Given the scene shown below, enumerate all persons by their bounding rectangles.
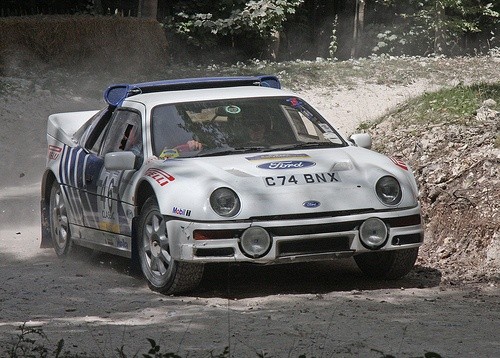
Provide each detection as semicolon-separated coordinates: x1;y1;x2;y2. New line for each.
229;106;275;151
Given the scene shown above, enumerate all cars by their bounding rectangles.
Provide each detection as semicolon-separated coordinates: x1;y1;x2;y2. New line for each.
40;77;425;295
